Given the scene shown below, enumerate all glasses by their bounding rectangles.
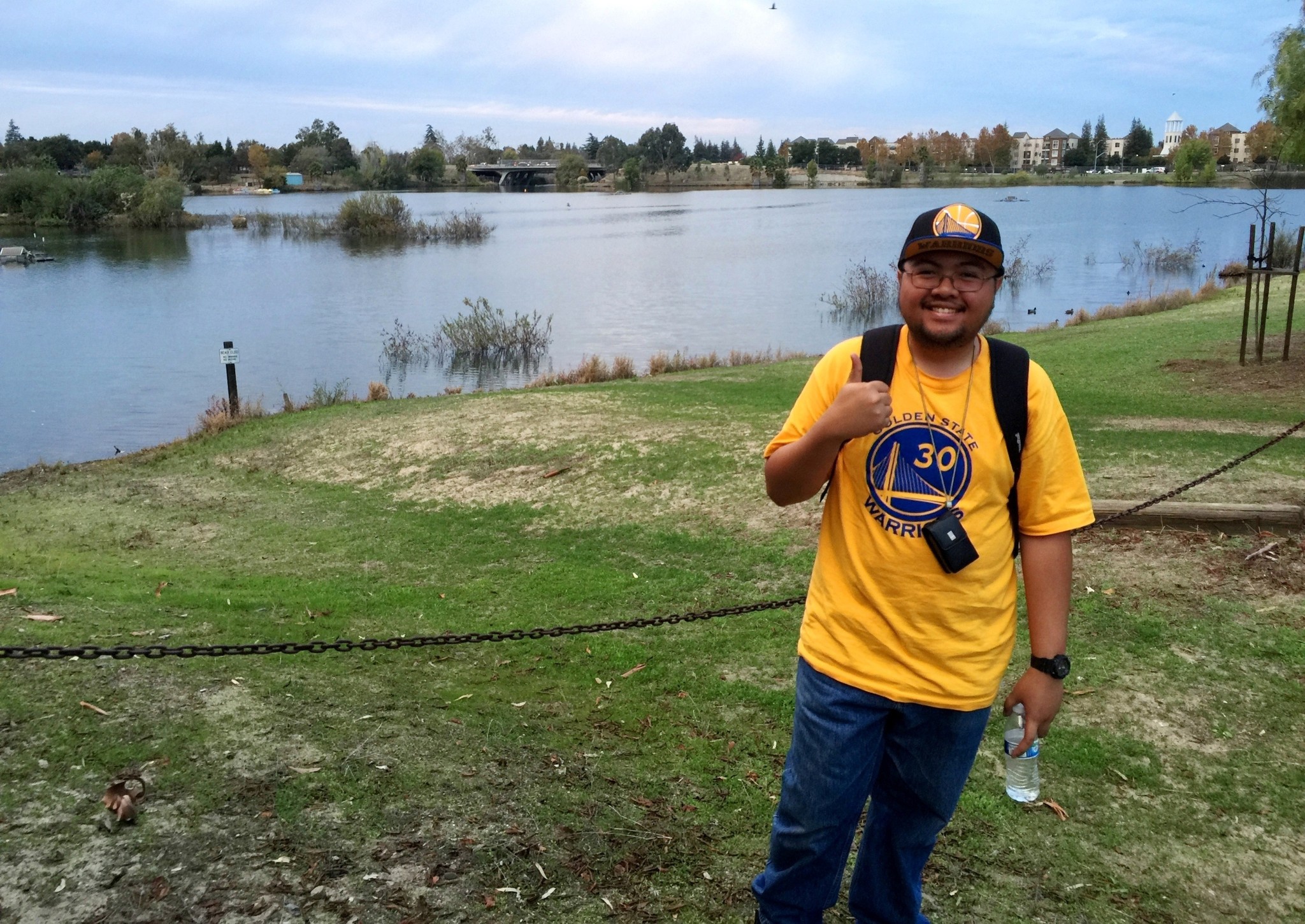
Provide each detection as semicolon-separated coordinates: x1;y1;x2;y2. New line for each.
902;261;999;292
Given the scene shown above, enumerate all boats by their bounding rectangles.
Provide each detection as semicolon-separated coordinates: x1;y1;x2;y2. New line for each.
231;188;280;194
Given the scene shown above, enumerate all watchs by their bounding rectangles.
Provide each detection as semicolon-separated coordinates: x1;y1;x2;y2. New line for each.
1030;652;1071;680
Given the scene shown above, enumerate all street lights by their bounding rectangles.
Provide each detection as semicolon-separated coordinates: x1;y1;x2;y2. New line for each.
1094;139;1103;170
1264;145;1267;168
497;141;499;160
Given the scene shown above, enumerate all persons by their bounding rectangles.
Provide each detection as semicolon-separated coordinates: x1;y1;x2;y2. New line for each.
750;203;1096;924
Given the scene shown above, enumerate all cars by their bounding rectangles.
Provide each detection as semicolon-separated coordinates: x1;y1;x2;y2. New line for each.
1096;169;1120;174
1086;169;1097;173
1243;167;1267;172
518;162;530;166
480;162;486;165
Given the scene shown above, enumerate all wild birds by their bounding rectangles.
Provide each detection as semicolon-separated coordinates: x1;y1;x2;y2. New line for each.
1028;307;1036;312
769;3;777;9
1202;264;1205;267
1066;309;1073;313
1127;291;1130;293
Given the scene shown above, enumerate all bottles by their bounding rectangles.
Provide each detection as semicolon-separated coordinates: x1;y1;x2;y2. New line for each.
1003;703;1040;802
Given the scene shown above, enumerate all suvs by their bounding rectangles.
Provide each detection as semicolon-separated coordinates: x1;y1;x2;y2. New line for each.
1146;167;1163;174
537;162;550;166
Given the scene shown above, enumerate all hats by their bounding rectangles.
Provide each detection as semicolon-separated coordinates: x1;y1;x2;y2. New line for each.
897;203;1004;272
